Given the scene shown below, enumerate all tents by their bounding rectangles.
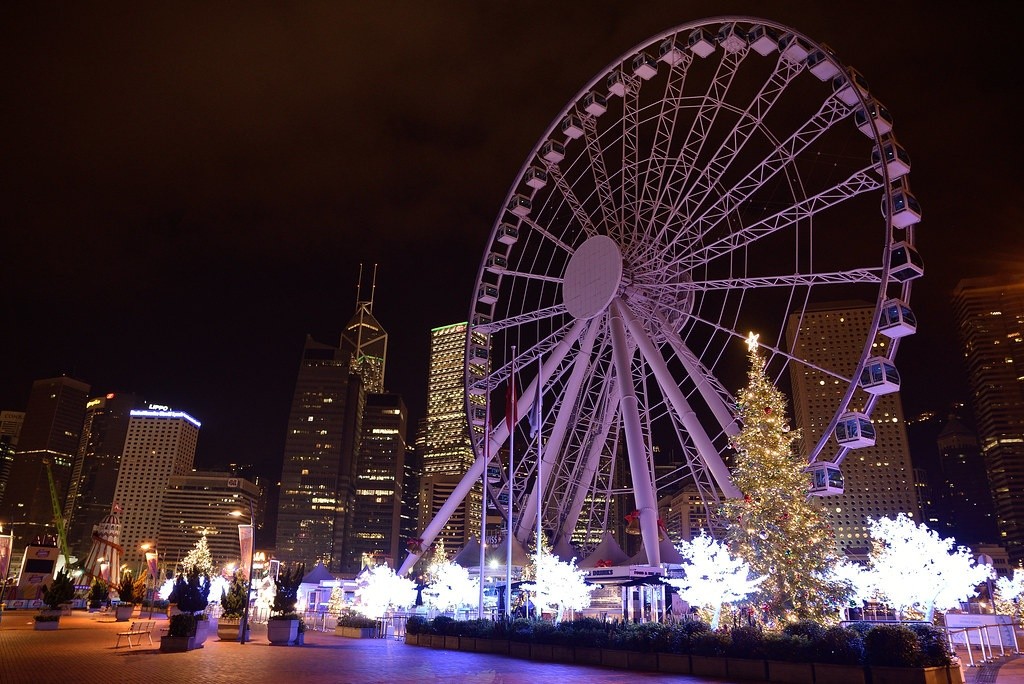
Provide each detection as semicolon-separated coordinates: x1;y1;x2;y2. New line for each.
290;532;692;627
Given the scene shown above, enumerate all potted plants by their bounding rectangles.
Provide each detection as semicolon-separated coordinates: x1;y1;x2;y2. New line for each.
33;562;379;652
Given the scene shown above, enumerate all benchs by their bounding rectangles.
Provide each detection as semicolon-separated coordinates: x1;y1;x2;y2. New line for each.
115;620;156;649
